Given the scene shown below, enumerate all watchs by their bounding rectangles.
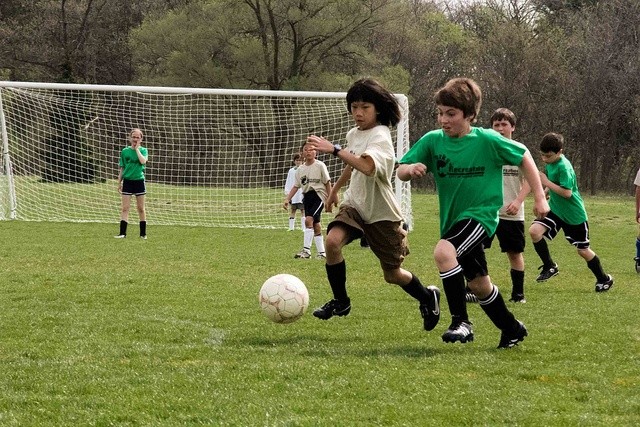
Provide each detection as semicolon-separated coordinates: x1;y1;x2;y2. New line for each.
333;144;341;156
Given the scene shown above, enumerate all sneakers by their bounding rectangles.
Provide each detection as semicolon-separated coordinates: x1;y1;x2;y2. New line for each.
114;235;125;238
497;319;528;348
509;292;526;303
316;253;326;258
442;315;473;342
536;262;560;282
295;249;311;259
313;298;351;319
634;257;640;272
140;234;147;239
419;285;440;331
595;274;613;292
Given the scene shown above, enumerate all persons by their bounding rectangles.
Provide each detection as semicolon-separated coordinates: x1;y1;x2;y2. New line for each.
396;79;551;349
283;142;333;260
529;132;614;292
465;108;532;302
312;77;441;331
284;153;306;232
634;168;640;273
113;128;149;240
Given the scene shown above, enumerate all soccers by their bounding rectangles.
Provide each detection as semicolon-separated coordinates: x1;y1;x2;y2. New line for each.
258;274;309;323
